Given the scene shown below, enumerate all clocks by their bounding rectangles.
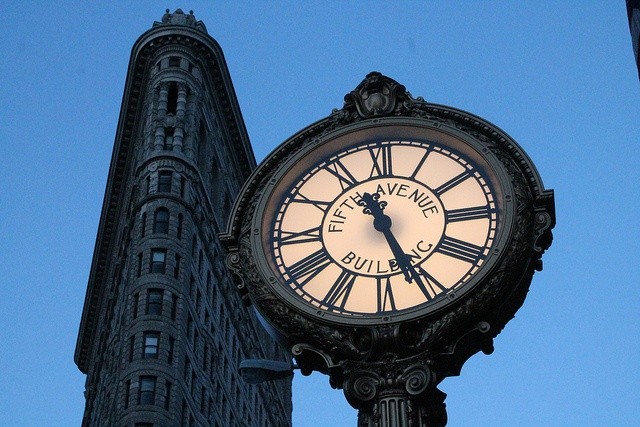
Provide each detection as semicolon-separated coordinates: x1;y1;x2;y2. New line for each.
251;114;518;326
